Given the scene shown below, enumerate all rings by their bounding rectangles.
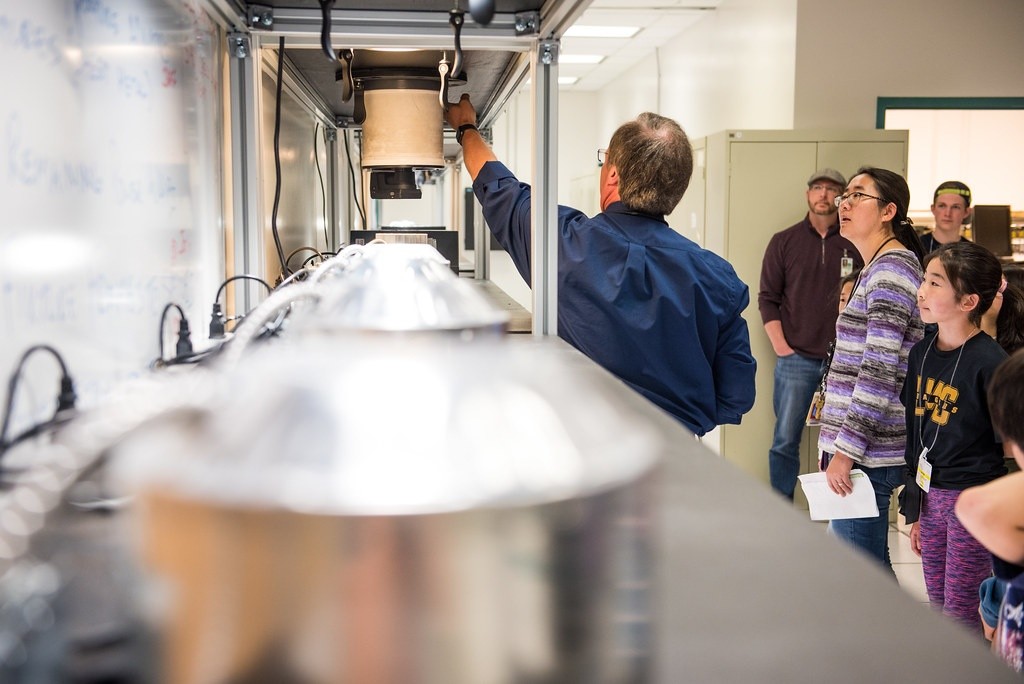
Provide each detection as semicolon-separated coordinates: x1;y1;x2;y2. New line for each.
839;483;842;485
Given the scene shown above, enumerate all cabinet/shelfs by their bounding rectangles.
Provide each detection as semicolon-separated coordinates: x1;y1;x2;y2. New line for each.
665;129;910;512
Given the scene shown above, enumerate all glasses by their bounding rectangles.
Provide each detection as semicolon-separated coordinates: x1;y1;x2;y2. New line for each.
834;192;889;207
598;149;609;162
809;184;843;195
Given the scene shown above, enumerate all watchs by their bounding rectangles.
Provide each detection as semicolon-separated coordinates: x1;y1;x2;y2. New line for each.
456;124;477;146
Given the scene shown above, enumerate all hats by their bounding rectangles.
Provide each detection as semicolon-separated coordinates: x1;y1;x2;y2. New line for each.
934;181;971;224
807;168;847;188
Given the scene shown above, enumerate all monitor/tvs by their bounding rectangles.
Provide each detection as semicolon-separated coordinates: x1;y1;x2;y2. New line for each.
971;205;1012;256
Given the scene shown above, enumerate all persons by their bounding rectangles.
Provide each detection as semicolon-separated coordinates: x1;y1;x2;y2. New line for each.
816;167;925;585
919;181;972;255
951;346;1024;674
758;167;865;505
898;242;1024;648
443;93;758;438
